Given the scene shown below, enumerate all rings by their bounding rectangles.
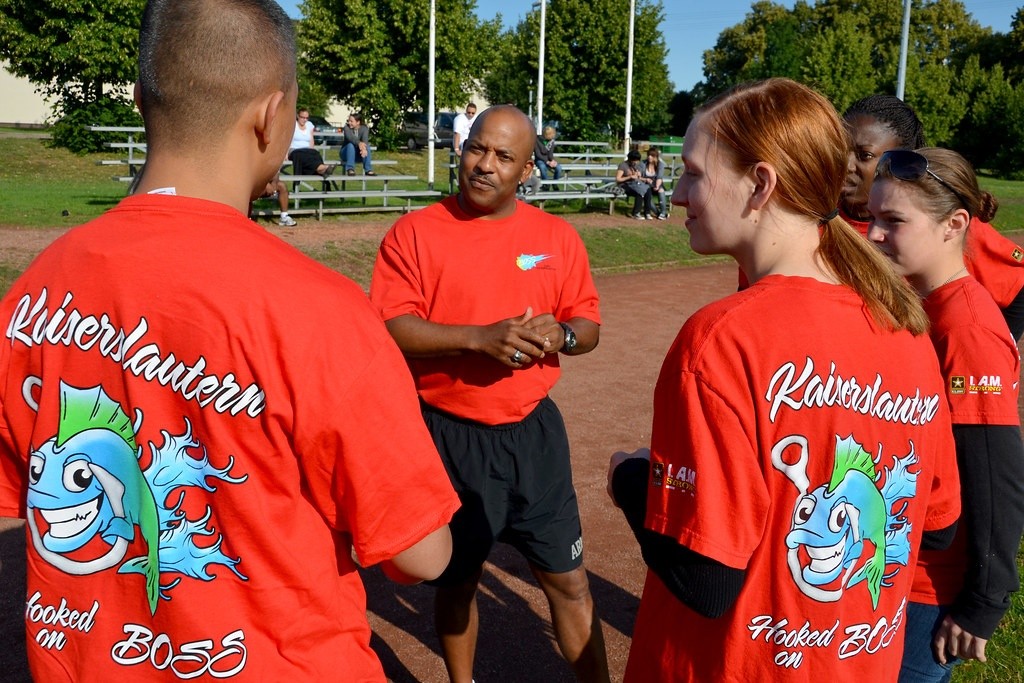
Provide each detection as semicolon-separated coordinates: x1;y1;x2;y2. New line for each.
514;351;522;362
544;335;549;342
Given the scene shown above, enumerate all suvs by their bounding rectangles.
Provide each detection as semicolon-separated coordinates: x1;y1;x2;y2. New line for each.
397;112;460;150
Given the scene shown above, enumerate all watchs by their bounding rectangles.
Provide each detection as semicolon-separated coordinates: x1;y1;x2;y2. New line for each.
559;322;577;353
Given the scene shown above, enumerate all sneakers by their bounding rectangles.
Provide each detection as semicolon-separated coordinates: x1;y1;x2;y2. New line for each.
279;215;297;226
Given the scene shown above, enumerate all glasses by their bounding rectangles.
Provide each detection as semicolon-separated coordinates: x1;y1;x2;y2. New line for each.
467;112;475;114
299;115;309;119
876;150;972;218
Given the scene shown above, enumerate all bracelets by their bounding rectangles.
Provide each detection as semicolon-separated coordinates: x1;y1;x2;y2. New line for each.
454;148;459;151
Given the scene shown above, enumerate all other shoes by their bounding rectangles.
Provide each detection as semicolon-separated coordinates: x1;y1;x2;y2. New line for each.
646;213;653;219
633;213;645;220
347;169;356;176
324;168;331;179
553;185;559;191
543;185;550;191
666;213;670;218
330;163;337;174
658;213;666;220
365;171;377;176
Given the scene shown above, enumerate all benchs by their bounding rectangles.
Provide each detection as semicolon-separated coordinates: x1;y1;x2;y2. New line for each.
88;123;684;222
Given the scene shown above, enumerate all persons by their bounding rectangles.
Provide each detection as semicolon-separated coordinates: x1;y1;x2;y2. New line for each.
1;0;612;683
288;107;336;193
606;77;1024;682
616;149;653;220
259;172;297;226
642;146;669;220
340;112;377;176
453;103;478;156
534;126;562;191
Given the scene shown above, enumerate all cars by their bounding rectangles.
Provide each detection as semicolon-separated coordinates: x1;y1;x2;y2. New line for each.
307;116;345;144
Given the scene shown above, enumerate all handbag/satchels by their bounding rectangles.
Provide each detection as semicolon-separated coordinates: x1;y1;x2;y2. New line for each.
628;179;650;198
